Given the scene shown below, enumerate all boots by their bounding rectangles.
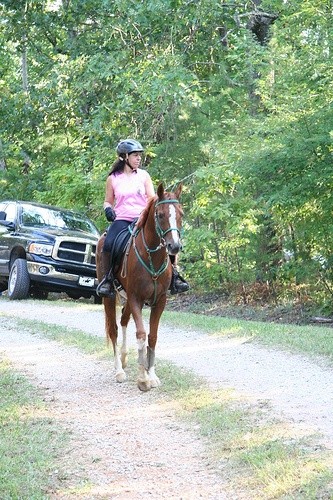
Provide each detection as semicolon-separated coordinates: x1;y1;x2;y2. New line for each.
169;254;189;294
99;252;111;295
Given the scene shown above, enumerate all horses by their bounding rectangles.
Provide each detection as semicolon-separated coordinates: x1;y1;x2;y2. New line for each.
96;183;184;391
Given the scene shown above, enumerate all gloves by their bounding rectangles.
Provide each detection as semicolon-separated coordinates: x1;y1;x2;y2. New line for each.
105;207;116;222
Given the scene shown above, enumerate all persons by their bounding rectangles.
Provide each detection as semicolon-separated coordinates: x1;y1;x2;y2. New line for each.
98;140;189;295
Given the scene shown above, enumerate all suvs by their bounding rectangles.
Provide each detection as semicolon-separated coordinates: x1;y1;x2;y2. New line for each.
0;200;106;305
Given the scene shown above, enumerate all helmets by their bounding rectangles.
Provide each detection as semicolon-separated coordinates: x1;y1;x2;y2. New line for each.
116;139;145;155
